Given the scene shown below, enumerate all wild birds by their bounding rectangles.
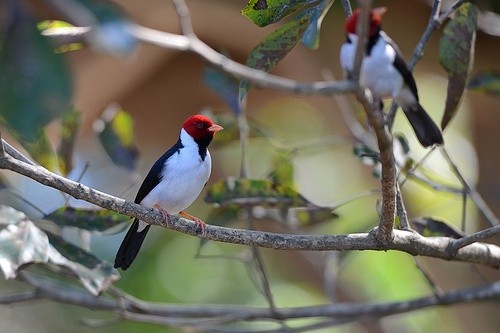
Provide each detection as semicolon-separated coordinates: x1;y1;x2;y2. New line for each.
113;113;224;271
337;6;445;146
438;2;479;131
